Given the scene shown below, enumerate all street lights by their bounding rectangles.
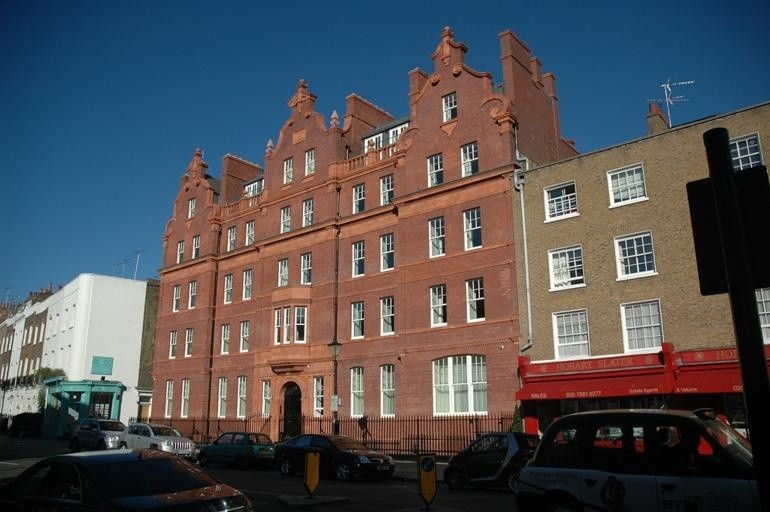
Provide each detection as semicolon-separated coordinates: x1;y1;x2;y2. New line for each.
328;336;341;436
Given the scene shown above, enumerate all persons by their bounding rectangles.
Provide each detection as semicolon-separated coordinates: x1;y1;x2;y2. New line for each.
359;411;372;444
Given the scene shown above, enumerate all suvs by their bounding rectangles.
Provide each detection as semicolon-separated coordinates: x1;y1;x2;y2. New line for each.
70;419;126;454
121;421;195;458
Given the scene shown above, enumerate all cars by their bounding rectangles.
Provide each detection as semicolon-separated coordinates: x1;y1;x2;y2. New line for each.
732;420;749;440
0;448;253;511
516;407;759;511
271;431;394;480
445;432;538;491
195;431;277;467
